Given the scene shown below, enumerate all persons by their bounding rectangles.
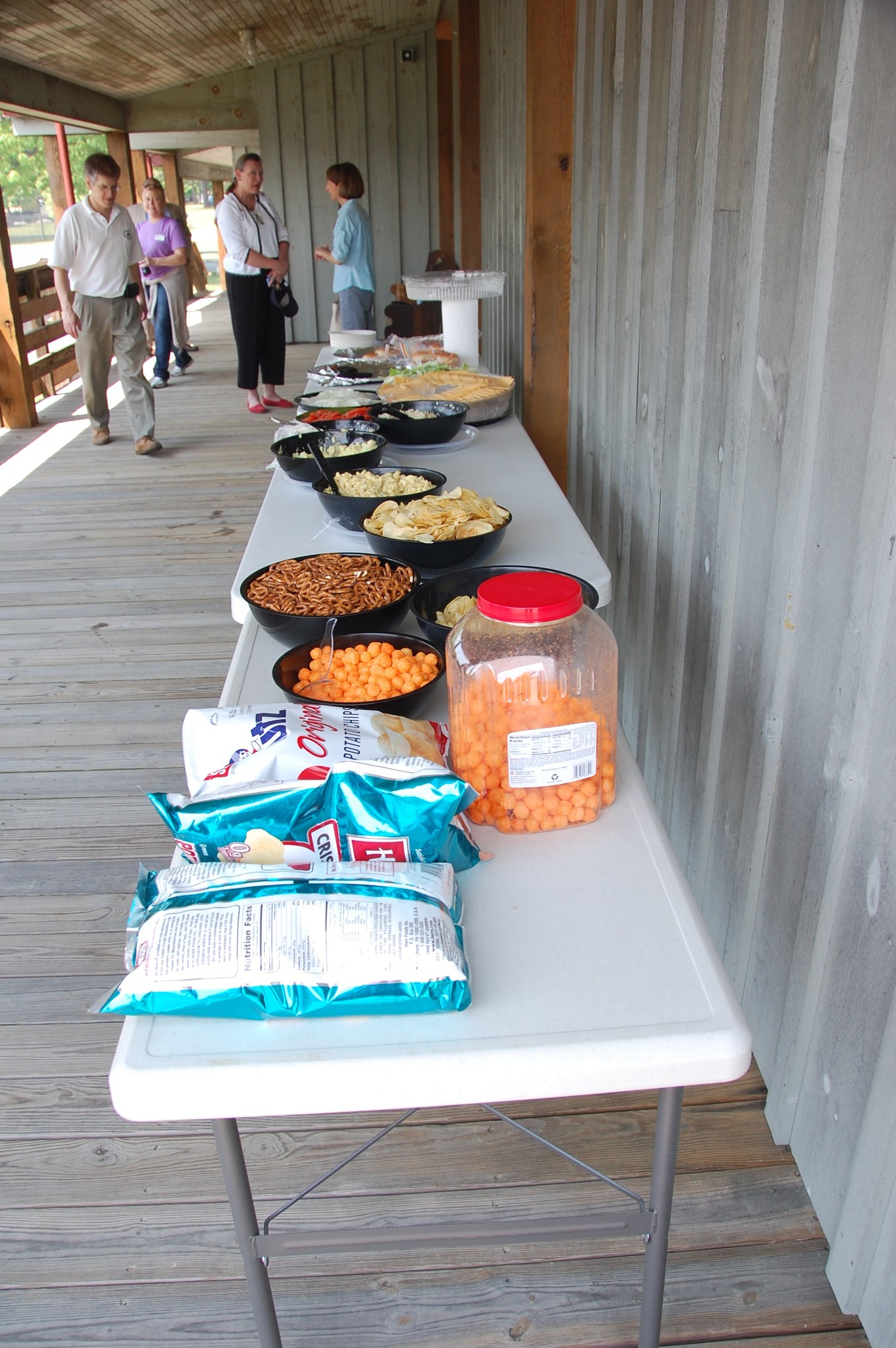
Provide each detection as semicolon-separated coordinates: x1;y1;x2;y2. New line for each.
214;176;238;252
133;185;196;391
47;152;165;454
313;160;378;341
217;152;295;413
125;177;199;356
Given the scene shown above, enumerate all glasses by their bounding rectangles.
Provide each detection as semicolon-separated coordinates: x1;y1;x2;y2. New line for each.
91;181;124;193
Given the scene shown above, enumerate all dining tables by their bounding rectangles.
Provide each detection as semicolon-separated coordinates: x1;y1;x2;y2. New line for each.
226;333;610;614
107;606;749;1348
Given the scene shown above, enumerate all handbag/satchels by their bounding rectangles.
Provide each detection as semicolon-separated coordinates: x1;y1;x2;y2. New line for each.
329;299;342;334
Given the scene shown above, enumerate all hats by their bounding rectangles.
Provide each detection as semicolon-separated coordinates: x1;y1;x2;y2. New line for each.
270;278;300;318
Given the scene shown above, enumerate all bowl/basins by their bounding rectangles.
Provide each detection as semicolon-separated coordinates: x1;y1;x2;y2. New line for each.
239;552;599;718
328;330;376;349
270;390;513;583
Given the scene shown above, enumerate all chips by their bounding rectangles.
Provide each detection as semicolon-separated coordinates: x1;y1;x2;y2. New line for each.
86;697;495;1030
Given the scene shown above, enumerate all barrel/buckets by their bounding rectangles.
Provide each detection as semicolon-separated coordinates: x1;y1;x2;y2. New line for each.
445;570;618;832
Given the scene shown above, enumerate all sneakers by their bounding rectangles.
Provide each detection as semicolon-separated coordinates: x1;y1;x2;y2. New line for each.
172;358;194;375
150;375;167;389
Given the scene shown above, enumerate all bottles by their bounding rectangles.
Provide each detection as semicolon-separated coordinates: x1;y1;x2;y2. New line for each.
141;267;151;276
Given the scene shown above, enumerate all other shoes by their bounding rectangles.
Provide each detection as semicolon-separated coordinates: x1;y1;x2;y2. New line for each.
247;398;266;413
262;393;293;408
92;425;110;445
148;347;156;357
135;436;162;456
180;342;197;351
